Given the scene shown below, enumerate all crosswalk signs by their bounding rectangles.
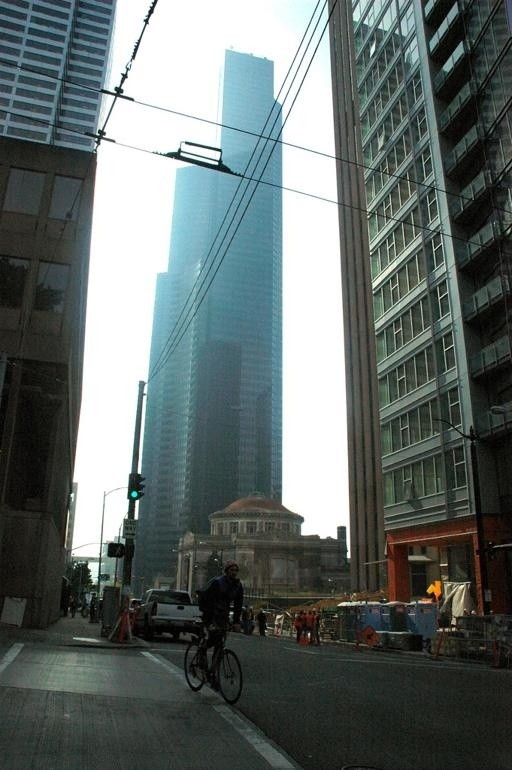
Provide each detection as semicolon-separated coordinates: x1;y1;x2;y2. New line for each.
108;543;124;558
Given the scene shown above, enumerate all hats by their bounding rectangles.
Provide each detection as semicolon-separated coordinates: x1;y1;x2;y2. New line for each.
224;559;240;574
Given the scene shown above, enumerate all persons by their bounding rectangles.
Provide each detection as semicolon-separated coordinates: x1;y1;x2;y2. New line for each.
241;605;321;647
68;592;99;625
195;558;245;691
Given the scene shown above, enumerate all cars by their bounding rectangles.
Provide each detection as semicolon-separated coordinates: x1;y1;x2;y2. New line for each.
229;606;257;632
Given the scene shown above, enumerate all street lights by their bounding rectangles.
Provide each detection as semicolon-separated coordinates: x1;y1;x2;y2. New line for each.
432;418;491;615
172;541;223;591
327;576;336;593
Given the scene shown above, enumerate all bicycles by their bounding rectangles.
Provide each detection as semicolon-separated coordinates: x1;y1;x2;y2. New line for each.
184;622;243;705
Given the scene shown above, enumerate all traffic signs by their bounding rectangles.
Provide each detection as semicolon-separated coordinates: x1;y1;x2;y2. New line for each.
122;519;137;539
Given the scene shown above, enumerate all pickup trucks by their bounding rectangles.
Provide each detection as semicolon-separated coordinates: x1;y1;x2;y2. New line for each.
129;589;204;640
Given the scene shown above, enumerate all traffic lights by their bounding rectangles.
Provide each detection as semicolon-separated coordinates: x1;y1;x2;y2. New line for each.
128;473;146;500
487;540;496;562
